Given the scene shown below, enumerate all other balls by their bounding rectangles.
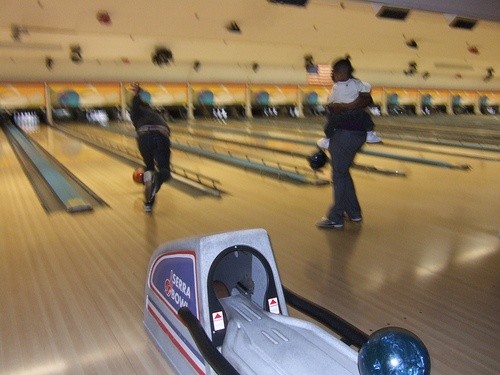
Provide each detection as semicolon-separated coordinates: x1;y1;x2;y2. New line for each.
133;168;145;185
357;326;431;375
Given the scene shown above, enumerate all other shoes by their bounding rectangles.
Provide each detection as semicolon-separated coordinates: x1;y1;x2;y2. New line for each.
366;130;382;143
316;137;330;149
143;170;157;202
144;205;152;212
344;211;363;221
317;219;343;229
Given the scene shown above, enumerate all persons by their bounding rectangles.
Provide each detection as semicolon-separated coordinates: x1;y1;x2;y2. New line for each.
127;81;174;212
317;59;382;150
316;55;374;228
